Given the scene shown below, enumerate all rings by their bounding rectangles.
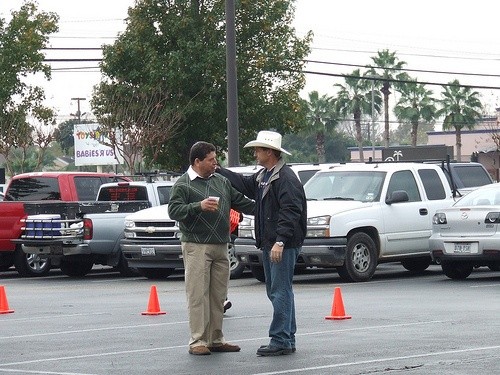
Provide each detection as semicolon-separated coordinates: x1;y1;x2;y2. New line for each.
275;255;279;258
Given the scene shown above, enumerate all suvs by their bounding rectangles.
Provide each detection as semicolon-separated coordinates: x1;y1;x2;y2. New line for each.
119;162;367;279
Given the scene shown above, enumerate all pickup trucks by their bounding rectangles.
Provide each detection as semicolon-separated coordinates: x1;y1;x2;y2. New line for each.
232;158;495;283
0;172;138;278
11;170;176;278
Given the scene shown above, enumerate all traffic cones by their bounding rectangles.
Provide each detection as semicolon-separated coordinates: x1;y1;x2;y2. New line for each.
0;285;15;314
324;287;351;320
141;285;166;316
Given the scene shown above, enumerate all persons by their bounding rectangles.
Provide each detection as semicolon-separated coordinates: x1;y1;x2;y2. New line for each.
168;141;257;355
213;130;307;355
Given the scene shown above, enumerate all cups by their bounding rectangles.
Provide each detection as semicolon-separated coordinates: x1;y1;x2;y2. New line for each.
209;196;219;208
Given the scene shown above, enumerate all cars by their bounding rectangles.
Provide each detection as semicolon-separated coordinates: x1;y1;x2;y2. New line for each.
428;184;500;280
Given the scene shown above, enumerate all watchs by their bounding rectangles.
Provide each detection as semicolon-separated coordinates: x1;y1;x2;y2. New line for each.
275;241;285;247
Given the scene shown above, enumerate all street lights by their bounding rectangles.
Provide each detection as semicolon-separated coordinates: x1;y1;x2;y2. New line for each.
364;74;382;162
71;98;87;124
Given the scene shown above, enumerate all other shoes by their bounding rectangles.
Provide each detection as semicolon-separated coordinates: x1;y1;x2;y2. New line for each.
188;345;211;355
207;343;241;352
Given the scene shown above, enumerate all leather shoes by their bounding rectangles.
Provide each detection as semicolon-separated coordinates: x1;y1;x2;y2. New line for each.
256;344;296;356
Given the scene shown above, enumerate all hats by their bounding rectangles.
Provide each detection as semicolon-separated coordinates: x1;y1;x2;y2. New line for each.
243;130;293;156
